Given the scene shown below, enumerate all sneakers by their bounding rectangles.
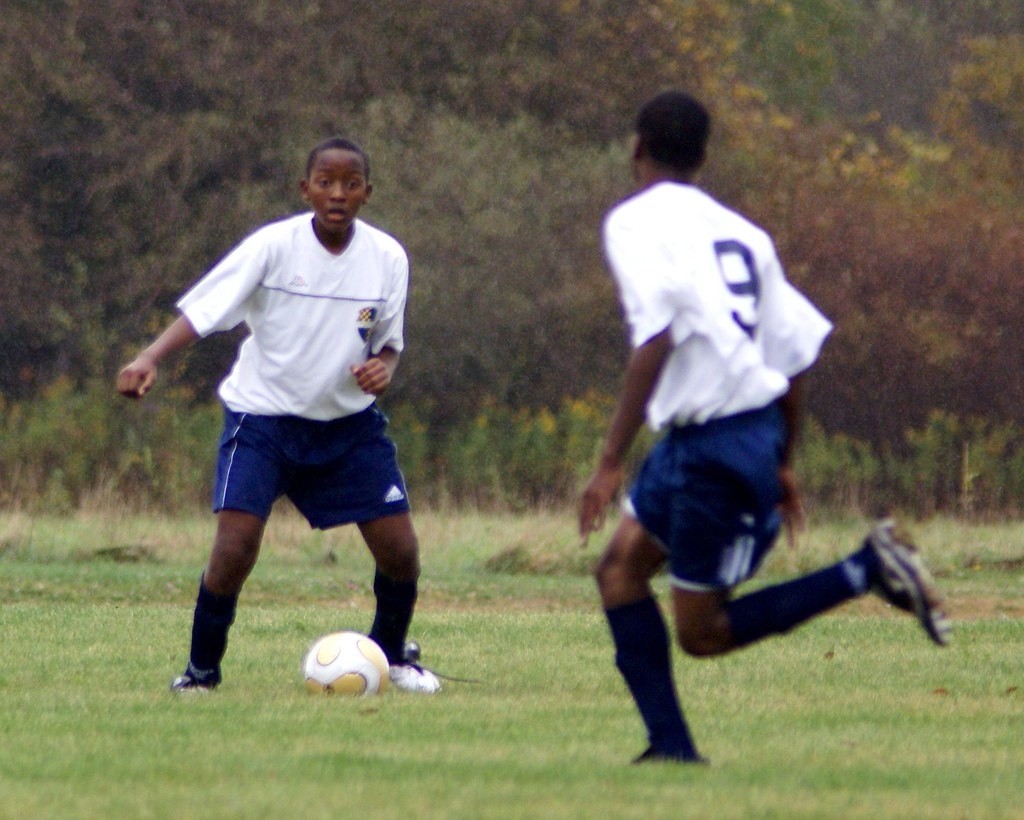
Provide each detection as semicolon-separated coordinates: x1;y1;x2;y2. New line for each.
864;519;955;646
388;659;439;692
171;663;219;690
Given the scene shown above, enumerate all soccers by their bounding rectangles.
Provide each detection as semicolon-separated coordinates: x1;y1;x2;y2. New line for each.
305;631;389;699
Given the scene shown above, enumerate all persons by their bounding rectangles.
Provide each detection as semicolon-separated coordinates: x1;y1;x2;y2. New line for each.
576;94;954;762
117;139;441;692
